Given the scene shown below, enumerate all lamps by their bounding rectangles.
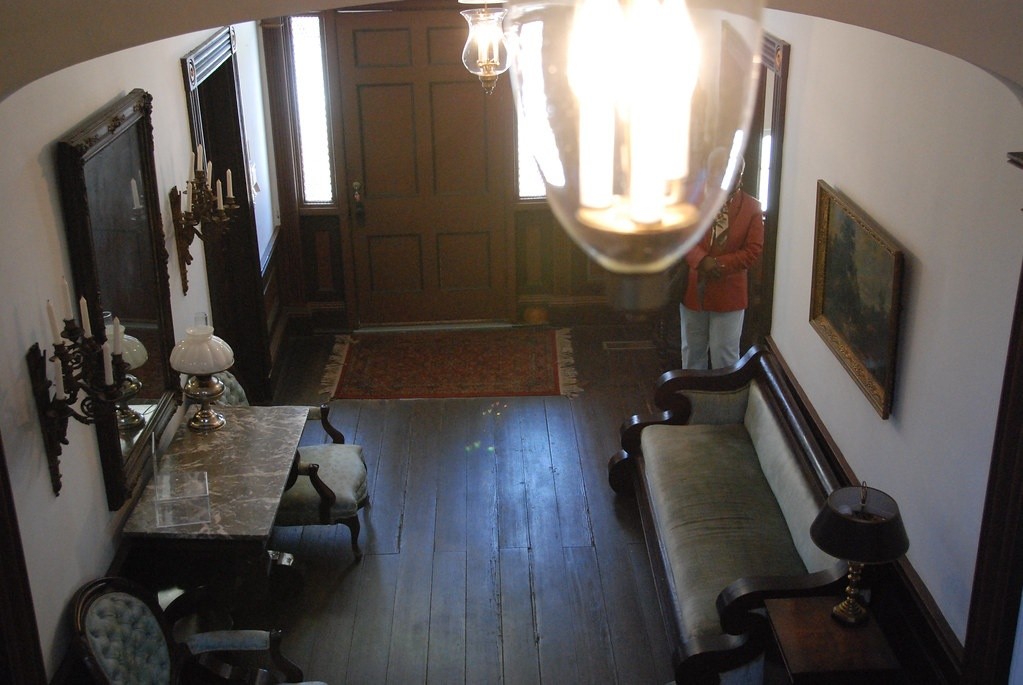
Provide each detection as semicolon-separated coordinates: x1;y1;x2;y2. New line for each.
169;322;236;433
808;481;911;629
100;312;148;431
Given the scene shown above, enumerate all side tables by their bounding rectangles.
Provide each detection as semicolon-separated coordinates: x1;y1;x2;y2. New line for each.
758;592;908;684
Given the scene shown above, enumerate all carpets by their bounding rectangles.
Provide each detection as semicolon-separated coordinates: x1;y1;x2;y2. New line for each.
315;325;579;404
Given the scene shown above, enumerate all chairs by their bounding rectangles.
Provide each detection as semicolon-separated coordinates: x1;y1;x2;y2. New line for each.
178;370;370;554
68;574;327;685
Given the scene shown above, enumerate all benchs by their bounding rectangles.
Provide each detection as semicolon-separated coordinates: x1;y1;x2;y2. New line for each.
608;348;892;685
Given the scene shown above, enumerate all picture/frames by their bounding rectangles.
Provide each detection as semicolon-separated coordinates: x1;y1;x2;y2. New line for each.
806;179;904;421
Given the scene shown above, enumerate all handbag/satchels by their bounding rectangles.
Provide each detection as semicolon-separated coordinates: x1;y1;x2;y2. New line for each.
665;257;690;315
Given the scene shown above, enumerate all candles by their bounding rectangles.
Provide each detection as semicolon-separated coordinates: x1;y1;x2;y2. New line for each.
55;356;65;400
188;143;213;192
80;295;92;338
226;168;233;198
112;315;122;355
101;338;114;385
216;179;224;210
186;181;193;212
131;176;140;208
47;298;62;344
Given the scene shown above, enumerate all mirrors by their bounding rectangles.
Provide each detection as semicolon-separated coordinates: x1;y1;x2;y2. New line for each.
48;86;171;344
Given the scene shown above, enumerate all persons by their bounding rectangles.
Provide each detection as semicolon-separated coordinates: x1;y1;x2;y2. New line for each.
679;147;764;369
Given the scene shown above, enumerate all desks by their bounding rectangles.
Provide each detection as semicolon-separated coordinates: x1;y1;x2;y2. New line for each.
117;402;311;614
